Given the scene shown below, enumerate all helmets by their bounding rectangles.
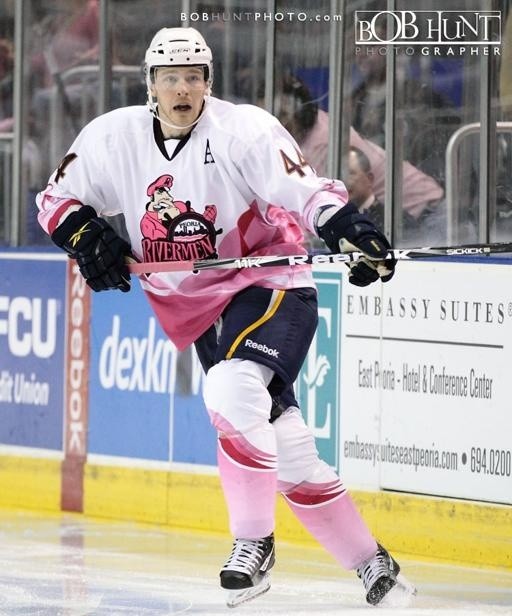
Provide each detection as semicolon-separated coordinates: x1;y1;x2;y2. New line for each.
141;28;216;101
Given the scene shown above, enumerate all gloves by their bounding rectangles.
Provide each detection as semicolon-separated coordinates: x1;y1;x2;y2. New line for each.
316;202;396;288
50;204;136;292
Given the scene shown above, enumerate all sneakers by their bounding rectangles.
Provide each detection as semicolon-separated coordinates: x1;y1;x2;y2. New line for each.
219;533;276;588
355;543;401;607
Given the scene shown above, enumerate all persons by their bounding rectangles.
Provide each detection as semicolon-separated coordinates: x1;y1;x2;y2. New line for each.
0;0;477;251
35;25;400;606
